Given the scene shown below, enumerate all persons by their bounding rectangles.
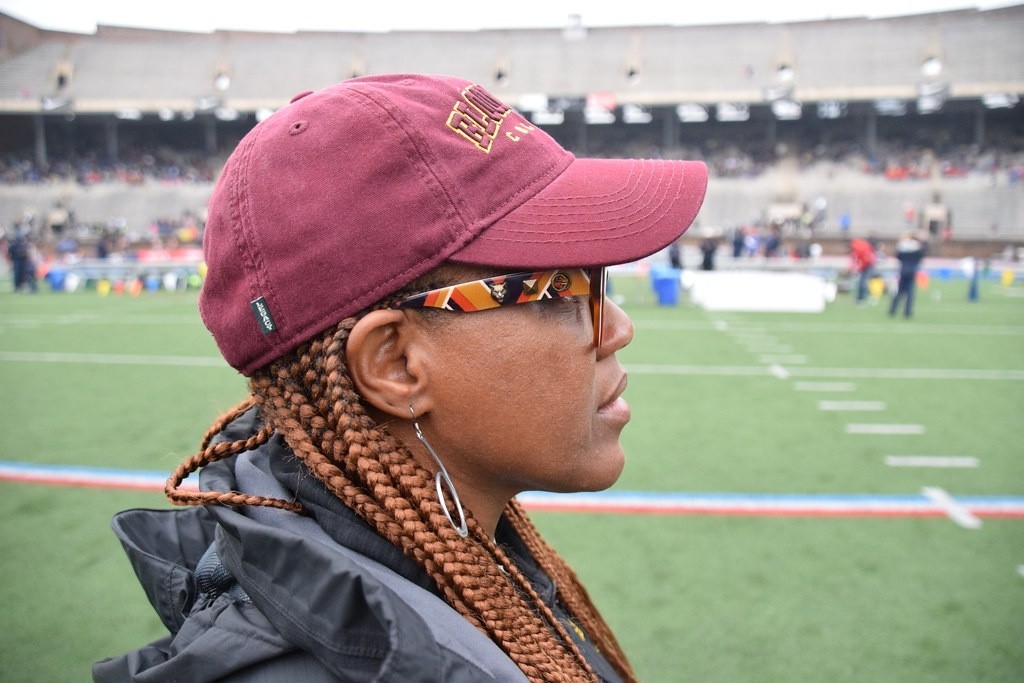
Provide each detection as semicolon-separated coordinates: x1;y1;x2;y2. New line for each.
0;120;1024;323
87;68;641;683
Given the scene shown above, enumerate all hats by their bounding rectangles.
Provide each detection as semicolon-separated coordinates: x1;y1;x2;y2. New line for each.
197;75;708;377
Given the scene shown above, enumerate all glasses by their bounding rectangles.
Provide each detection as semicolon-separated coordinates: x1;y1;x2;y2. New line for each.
388;267;606;345
